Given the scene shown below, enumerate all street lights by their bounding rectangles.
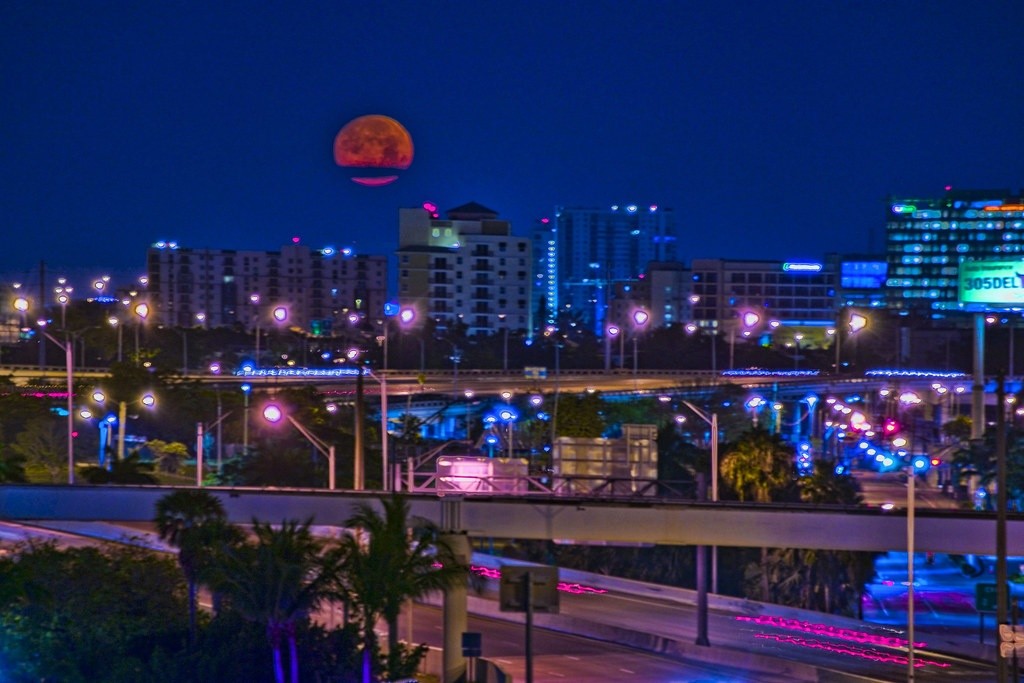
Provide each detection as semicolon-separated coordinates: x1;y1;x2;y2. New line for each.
383;308;416;492
264;404;339;489
196;405;259;486
659;393;718;594
18;322;77;486
93;390;155;462
